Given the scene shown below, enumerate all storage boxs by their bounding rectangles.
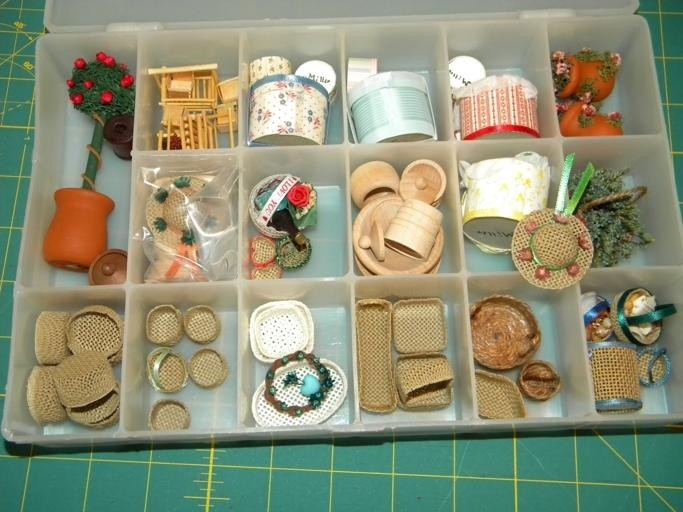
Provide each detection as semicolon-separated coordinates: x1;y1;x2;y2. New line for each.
1;0;682;447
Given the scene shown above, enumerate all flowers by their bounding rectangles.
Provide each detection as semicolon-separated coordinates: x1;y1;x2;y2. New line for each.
287;183;312;210
549;45;622;104
552;100;624;131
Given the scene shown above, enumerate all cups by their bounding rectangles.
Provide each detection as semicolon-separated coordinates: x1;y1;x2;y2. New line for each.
382;199;444;262
87;249;126;285
102;114;132;160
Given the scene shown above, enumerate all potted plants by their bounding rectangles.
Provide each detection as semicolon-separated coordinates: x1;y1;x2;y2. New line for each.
39;50;136;272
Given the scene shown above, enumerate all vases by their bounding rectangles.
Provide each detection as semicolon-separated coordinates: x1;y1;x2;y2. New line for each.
556;100;623;137
555;53;616;104
